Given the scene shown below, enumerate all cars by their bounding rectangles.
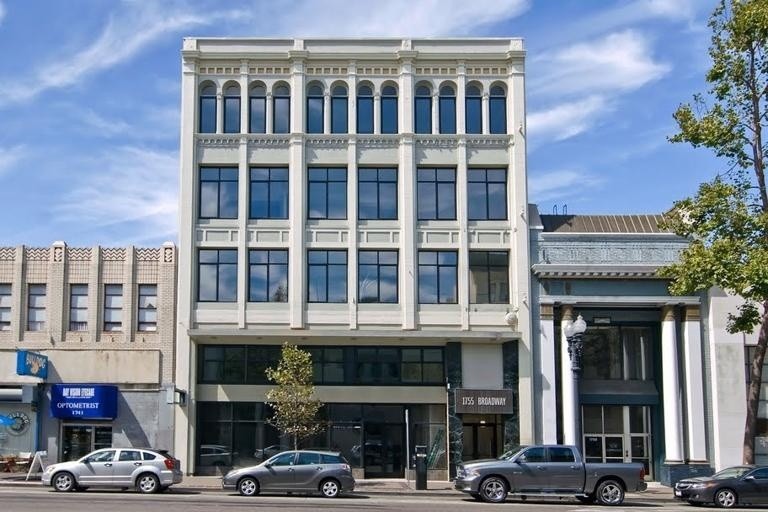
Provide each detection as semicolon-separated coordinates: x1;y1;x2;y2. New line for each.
199;445;240;474
41;447;183;494
674;464;768;508
413;445;462;467
254;444;289;459
352;443;393;465
222;450;355;498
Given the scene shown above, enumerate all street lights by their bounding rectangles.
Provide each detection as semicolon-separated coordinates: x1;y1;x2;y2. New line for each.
564;312;587;445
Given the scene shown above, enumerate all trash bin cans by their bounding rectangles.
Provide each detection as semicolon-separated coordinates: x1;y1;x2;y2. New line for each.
415;445;428;490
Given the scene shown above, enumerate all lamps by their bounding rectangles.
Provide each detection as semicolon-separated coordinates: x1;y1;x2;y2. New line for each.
480;420;485;429
593;316;611;324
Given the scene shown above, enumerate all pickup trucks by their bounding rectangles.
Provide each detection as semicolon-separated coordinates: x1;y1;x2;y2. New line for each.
454;444;647;506
